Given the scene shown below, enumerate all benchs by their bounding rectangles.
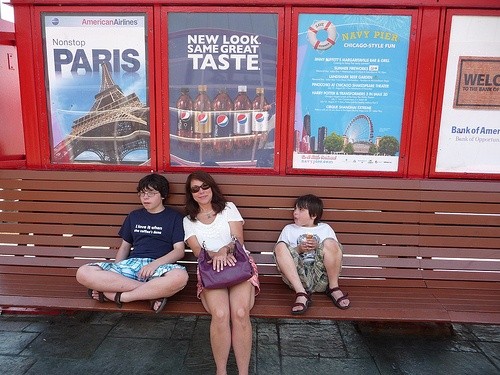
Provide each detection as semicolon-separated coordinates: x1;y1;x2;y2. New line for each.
0;168;500;326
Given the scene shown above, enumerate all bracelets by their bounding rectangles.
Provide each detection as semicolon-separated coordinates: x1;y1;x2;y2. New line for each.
224;245;230;253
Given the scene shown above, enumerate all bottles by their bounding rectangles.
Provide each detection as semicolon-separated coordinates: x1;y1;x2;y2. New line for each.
234;86;252;149
212;87;234;153
251;88;268;135
193;84;212;151
176;88;193;150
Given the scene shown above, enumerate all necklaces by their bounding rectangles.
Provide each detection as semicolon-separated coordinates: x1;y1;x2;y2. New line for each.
205;209;213;219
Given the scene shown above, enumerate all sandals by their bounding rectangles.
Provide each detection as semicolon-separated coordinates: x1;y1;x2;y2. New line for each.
88;288;123;308
291;291;312;315
326;284;351;310
150;297;167;314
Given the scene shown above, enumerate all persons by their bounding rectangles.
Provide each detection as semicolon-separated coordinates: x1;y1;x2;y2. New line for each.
272;194;351;314
183;171;260;375
76;174;189;314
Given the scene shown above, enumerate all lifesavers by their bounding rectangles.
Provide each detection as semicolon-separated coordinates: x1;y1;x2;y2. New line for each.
307;20;336;50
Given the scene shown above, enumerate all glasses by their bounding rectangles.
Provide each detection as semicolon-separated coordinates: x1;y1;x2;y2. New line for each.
191;181;210;193
137;191;158;197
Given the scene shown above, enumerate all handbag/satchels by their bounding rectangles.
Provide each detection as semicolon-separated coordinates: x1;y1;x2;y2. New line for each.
198;238;254;289
